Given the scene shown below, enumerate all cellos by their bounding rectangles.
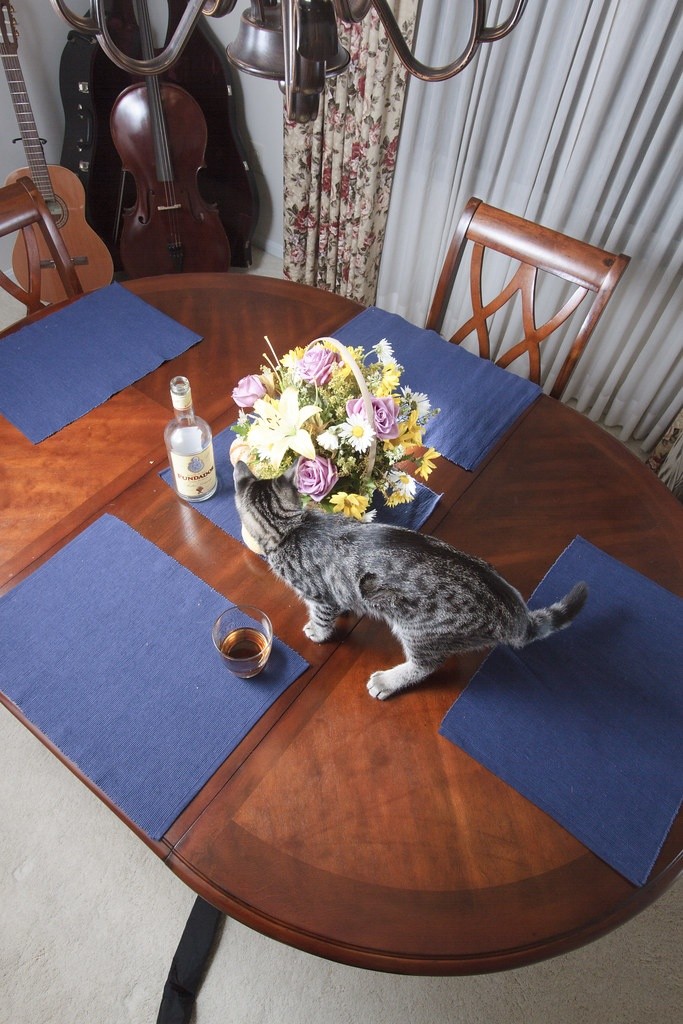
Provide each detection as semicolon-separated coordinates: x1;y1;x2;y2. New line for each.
110;0;231;280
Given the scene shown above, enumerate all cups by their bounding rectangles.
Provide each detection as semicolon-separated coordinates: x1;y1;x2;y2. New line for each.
212;604;273;679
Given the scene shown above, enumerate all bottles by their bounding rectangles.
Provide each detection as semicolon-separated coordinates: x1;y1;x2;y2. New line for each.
163;375;218;503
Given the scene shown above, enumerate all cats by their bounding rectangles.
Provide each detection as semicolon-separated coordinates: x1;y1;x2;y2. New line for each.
232;454;588;701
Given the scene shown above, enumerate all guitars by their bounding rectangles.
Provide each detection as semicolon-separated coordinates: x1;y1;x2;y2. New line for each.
0;0;114;304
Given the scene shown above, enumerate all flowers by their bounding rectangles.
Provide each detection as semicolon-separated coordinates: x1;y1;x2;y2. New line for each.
230;334;441;522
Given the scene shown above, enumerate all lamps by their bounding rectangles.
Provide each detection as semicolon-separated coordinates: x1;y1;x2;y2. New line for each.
49;1;532;127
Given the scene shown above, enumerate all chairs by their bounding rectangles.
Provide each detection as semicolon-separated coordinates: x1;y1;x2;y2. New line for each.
423;195;632;402
0;175;84;333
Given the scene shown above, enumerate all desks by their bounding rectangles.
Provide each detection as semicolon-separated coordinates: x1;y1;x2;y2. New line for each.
0;274;682;1023
